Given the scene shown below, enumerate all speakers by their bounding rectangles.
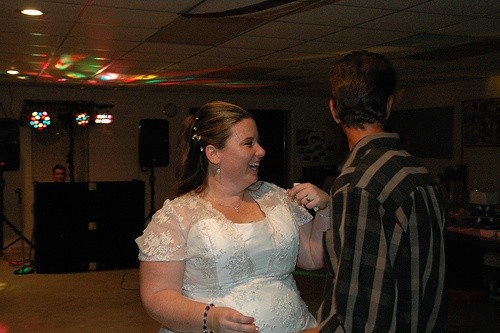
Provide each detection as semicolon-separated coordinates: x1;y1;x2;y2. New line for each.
138;119;170;168
0;117;20;172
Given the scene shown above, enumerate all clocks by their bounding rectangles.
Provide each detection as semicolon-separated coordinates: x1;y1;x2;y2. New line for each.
164;103;177;117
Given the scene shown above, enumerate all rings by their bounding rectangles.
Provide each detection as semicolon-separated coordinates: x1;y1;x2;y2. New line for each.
306;197;311;203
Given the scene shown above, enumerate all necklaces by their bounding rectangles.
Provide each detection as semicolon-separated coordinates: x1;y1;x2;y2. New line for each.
204;190;244;213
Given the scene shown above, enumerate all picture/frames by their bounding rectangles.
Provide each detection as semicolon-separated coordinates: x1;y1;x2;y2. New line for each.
461;97;500;148
297;130;325;161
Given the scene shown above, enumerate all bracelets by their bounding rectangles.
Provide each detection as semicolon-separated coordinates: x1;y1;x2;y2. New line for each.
202;303;215;333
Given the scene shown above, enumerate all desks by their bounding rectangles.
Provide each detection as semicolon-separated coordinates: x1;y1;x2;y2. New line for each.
447;226;500;257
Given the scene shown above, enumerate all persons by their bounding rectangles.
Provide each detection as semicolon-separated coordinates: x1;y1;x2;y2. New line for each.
52;164;66;182
135;101;331;333
301;50;447;333
